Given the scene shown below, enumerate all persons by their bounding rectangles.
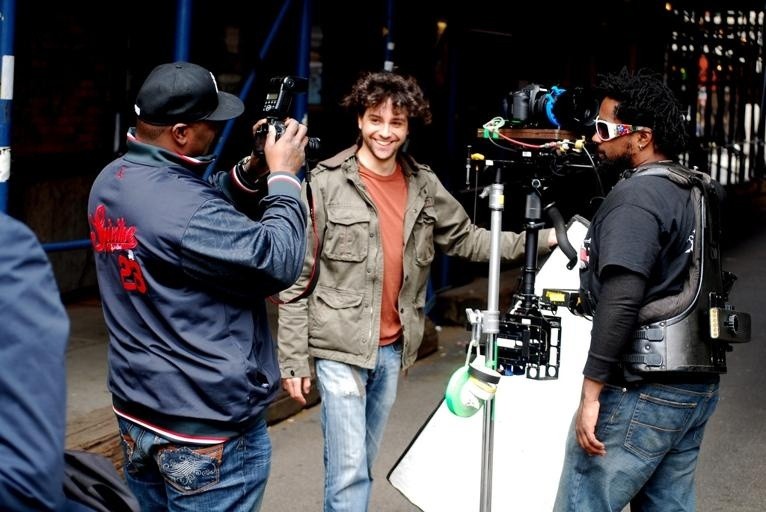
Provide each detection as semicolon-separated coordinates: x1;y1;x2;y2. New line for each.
87;60;312;512
0;212;140;510
274;71;567;511
553;60;733;510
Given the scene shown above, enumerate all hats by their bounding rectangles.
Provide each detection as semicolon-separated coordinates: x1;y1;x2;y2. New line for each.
132;59;245;128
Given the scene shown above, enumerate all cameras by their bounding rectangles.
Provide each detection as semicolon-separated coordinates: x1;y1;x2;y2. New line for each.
252;77;321;156
502;83;600;129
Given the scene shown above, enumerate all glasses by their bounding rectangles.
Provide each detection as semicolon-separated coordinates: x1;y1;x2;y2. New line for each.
593;114;652;143
